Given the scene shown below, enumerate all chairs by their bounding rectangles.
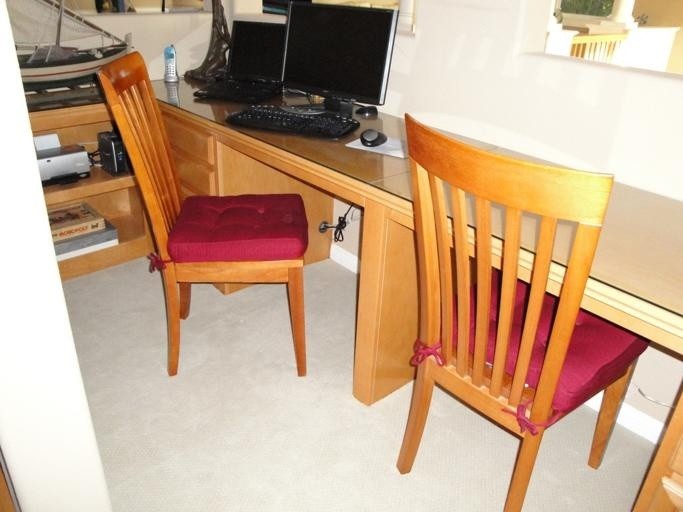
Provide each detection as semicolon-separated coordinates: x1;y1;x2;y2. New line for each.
96;51;310;378
395;111;649;511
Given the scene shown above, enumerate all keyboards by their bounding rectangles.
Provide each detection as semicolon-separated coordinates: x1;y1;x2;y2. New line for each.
225;105;360;140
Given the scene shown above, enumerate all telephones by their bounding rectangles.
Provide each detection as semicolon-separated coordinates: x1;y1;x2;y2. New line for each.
164;44;178;82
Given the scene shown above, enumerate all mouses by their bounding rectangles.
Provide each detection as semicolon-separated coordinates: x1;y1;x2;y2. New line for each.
360;129;387;148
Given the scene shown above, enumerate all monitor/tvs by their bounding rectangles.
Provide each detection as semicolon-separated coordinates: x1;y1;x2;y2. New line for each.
280;0;400;115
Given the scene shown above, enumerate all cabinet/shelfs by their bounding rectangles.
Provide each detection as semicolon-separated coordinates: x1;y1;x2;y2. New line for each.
27;101;155;282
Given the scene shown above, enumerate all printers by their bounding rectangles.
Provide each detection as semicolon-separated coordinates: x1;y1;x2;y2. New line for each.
33;133;91;186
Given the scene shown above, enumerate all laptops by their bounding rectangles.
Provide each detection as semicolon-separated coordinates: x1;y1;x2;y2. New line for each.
194;20;285;105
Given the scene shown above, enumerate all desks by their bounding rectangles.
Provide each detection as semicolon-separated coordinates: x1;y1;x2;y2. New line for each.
147;73;499;407
364;150;682;510
